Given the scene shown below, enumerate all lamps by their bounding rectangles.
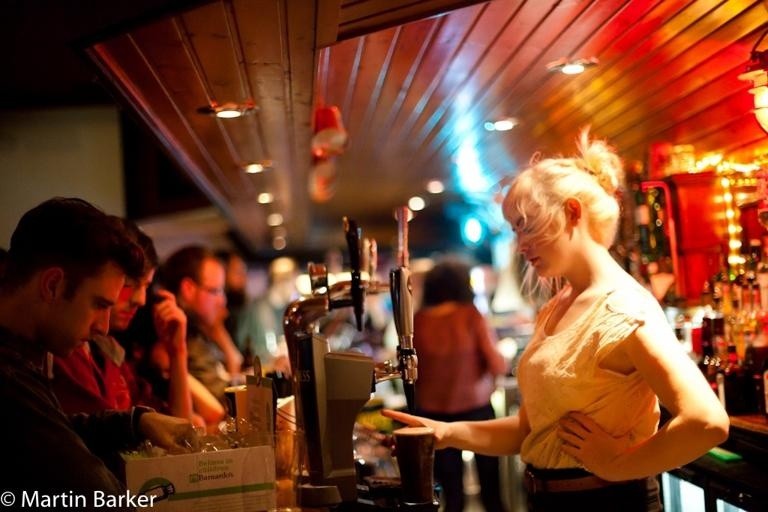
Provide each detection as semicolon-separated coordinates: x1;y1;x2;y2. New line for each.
310;106;347;159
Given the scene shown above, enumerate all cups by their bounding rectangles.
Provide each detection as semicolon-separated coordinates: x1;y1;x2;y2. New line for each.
392;425;435;506
274;429;303;511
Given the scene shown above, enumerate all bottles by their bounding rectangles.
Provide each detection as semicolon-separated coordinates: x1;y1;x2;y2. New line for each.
697;268;768;421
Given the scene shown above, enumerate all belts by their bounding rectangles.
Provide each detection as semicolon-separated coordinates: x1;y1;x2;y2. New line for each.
522;469;628;494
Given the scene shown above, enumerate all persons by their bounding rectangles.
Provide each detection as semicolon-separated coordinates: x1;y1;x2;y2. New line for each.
380;122;733;511
1;196;514;511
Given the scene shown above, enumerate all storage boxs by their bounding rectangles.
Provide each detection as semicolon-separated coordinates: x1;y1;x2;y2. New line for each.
124;434;277;512
